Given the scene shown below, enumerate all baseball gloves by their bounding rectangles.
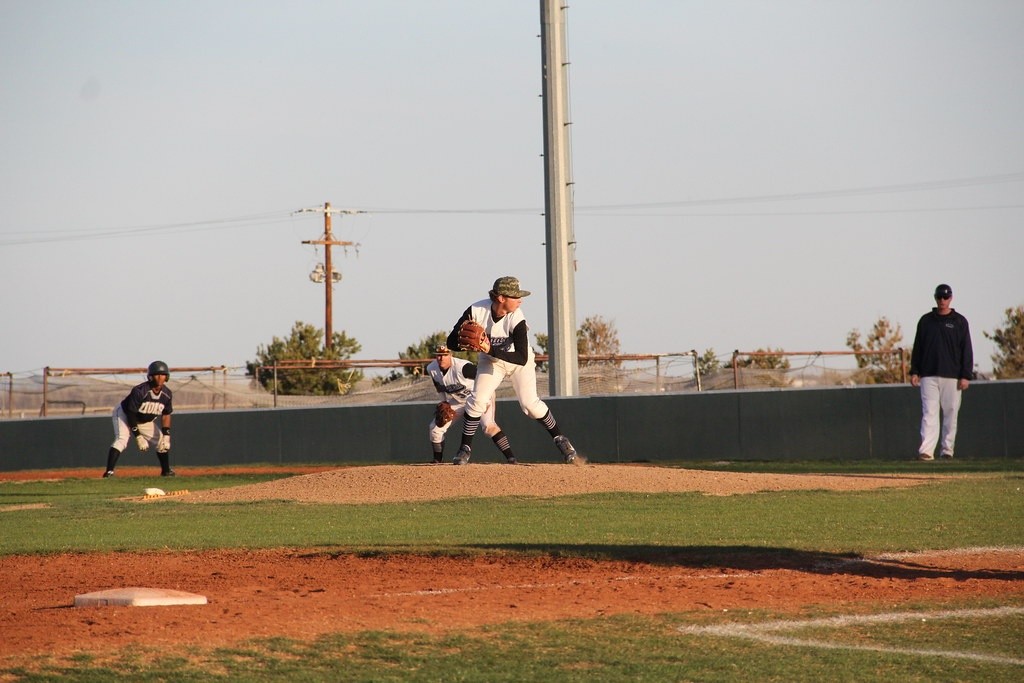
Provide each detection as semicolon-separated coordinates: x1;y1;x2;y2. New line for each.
457;313;492;355
433;400;456;427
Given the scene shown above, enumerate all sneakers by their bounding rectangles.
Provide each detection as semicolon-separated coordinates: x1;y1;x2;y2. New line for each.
453;443;471;464
552;435;576;463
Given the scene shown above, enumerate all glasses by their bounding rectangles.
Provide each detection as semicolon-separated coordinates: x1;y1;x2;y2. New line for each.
936;293;951;301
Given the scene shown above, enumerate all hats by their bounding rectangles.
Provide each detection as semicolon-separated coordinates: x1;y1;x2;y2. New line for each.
433;342;451;354
935;284;953;297
493;275;531;298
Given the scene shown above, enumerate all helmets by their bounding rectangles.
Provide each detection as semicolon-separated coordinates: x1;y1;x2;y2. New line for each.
148;361;170;382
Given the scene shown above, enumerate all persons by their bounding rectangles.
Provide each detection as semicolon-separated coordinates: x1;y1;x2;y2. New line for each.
910;284;973;460
427;346;516;464
446;276;576;464
103;361;176;477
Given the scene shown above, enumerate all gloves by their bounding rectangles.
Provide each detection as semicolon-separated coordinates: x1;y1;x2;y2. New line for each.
162;435;171;450
136;436;149;450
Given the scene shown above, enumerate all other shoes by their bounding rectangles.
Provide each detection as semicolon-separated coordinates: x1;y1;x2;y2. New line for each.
918;454;931;463
103;470;113;478
508;457;518;466
162;469;179;478
426;458;444;464
942;454;951;460
